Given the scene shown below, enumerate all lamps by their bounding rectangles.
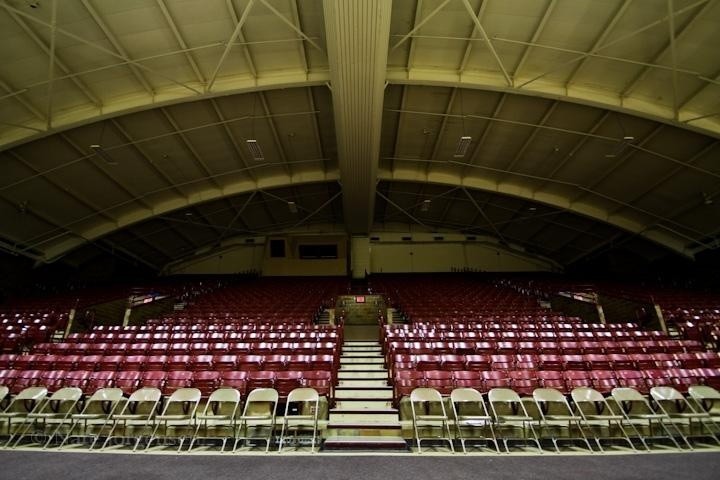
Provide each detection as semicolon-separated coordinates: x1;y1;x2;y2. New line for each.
288;132;299;214
91;105;119;166
604;93;635;159
454;69;472;158
422;130;431;212
246;76;266;162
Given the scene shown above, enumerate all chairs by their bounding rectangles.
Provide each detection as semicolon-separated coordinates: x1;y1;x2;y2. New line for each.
367;271;720;452
0;274;351;450
